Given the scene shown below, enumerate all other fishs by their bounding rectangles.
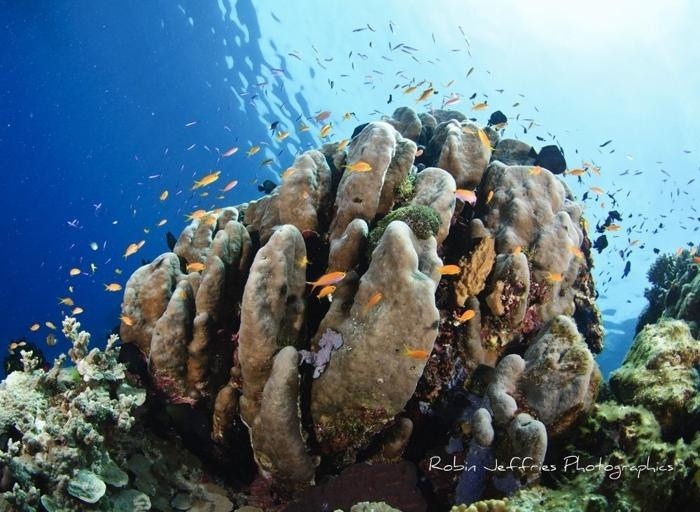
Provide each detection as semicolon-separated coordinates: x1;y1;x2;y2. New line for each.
6;13;700;371
